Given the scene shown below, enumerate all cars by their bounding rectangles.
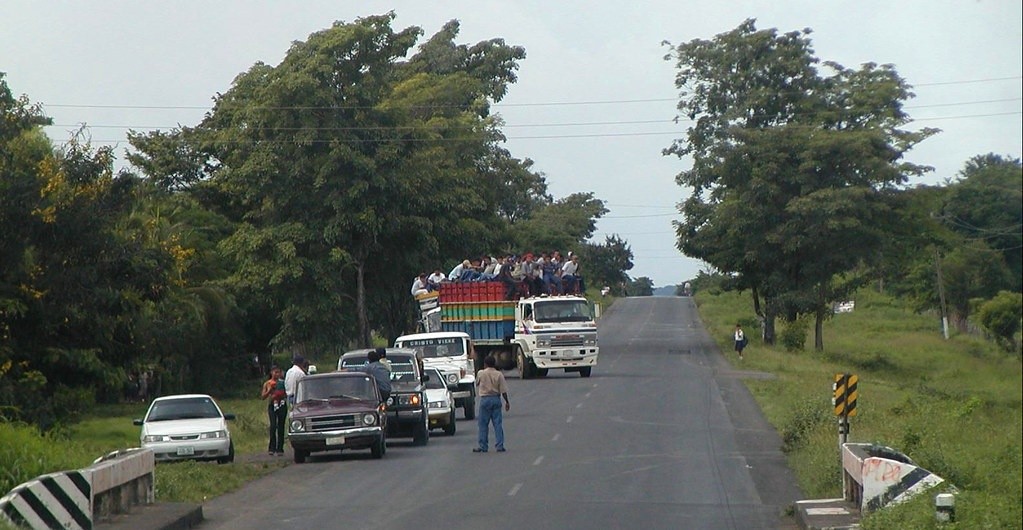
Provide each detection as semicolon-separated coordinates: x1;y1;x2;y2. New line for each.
287;371;394;464
133;394;236;465
389;366;459;437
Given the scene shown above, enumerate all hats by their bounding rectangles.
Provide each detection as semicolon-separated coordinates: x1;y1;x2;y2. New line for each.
292;355;304;364
526;254;533;258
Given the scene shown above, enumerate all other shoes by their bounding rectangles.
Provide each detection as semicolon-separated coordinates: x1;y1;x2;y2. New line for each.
269;451;274;455
497;449;506;452
505;298;513;301
277;452;283;456
473;448;487;452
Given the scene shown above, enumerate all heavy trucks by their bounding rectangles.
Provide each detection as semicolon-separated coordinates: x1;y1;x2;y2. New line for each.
439;293;602;380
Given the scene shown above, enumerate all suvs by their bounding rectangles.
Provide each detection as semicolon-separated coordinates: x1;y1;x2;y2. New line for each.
337;347;431;447
391;330;481;421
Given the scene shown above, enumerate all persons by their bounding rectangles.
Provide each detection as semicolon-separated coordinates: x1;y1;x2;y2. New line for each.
684;282;691;297
284;355;306;405
620;274;629;297
260;367;287;456
338;346;393;401
128;366;158;404
410;249;587;301
473;354;511;454
734;324;748;360
